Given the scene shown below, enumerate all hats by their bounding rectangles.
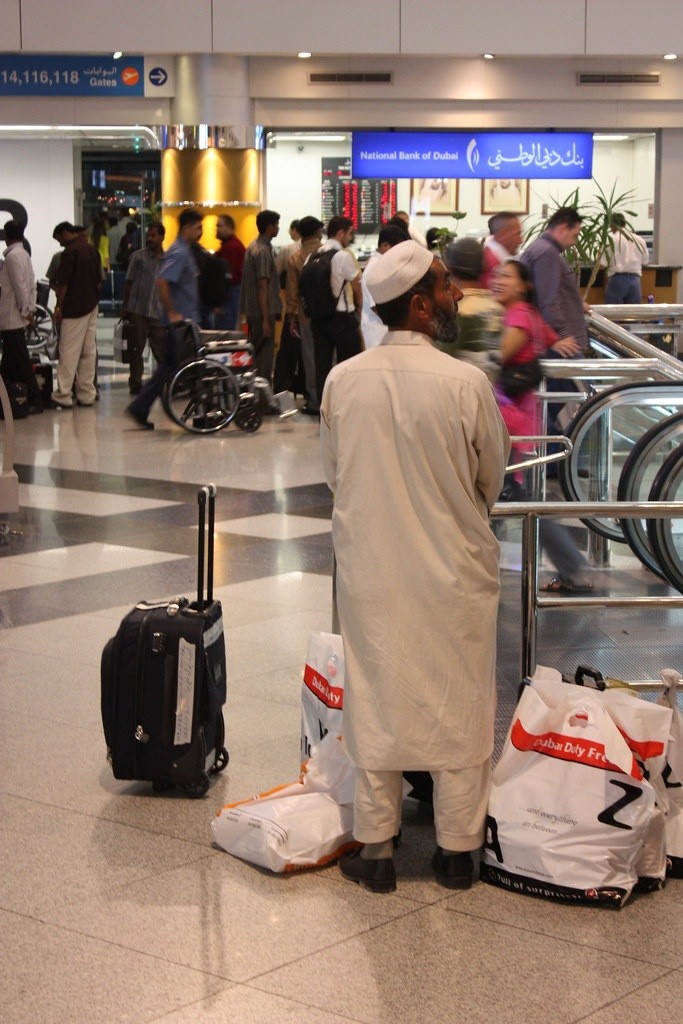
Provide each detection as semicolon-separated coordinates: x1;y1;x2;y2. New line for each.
365;239;434;304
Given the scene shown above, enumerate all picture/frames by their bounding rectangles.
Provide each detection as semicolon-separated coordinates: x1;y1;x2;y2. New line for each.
410;178;459;215
481;179;529;214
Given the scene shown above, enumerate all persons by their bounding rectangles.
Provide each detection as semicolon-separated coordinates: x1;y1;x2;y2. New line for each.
490;259;549;484
360;211;412;350
238;210;283;417
319;240;512;894
443;237;507;351
51;222;103;408
275;216;362;415
126;208;204;431
87;223;110;274
46;224;99;386
600;213;649;304
124;222;166;395
118;223;142;263
0;220;45;420
519;208;593;479
480;212;523;289
213;214;246;330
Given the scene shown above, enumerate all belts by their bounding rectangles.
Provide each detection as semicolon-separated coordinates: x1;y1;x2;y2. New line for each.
615;272;639;277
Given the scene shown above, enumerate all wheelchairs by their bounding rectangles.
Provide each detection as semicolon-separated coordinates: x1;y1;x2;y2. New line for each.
23;278;54;352
158;317;304;434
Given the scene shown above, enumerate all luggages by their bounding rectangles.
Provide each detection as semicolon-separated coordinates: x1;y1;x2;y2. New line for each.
100;482;226;800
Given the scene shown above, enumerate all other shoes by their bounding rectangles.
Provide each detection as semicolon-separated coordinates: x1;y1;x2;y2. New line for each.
130;385;143;394
45;398;72;409
301;405;319;416
28;405;43;414
125;408;154;429
76;399;92;406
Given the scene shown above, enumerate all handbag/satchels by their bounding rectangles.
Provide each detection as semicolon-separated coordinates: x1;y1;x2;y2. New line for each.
32;364;52;400
209;630;414;873
480;663;683;909
113;316;136;364
0;382;29;419
499;358;543;396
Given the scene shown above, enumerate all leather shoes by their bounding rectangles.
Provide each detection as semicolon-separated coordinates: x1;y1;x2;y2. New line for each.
432;847;474;889
337;847;397;893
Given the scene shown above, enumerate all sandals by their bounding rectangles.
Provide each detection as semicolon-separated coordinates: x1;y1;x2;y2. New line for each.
539;576;591;592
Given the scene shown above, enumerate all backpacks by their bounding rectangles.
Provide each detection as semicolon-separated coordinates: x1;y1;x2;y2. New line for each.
298;249;348;320
195;243;229;307
